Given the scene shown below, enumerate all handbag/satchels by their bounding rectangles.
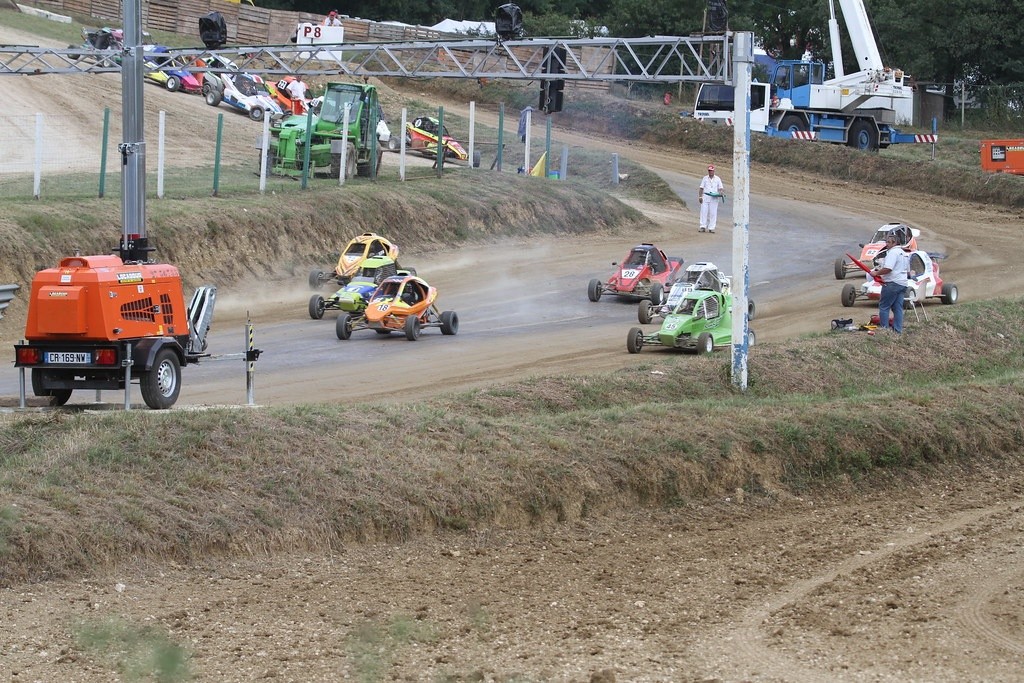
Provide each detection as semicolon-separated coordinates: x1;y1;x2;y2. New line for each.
831;319;853;330
867;315;893;325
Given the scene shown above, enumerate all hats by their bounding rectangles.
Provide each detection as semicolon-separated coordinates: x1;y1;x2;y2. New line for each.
708;166;715;170
330;11;336;16
639;253;646;258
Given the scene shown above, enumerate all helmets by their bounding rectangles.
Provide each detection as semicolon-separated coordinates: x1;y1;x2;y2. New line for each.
405;283;412;292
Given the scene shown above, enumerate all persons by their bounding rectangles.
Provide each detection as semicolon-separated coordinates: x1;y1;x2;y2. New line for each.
870;235;908;334
285;75;306;116
699;165;723;233
324;12;343;26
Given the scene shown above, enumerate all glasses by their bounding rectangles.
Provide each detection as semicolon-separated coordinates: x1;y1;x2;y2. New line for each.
708;170;713;171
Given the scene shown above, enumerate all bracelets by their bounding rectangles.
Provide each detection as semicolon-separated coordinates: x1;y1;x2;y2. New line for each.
699;197;703;198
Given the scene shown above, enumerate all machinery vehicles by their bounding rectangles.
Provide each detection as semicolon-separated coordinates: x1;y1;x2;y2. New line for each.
694;0;939;160
14;0;264;410
256;80;392;179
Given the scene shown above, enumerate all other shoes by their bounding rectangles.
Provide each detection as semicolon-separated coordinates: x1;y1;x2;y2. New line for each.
699;228;705;232
709;230;715;233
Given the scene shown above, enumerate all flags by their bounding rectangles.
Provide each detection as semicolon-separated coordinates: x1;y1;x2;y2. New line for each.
847;252;883;287
